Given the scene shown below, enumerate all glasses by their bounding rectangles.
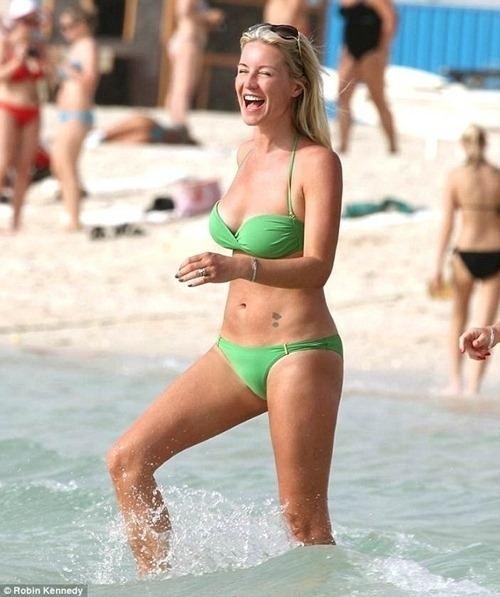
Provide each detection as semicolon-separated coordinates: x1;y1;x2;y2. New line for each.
242;22;299;51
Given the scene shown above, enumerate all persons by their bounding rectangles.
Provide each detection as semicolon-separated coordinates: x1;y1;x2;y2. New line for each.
1;0;50;235
432;124;500;398
457;319;500;361
166;0;226;123
110;24;342;579
49;4;103;232
262;0;314;40
336;0;400;154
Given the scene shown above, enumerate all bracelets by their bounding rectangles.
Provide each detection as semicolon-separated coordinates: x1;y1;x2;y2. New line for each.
488;324;495;349
251;255;258;280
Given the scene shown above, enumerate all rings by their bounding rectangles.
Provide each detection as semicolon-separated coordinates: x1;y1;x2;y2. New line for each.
199;267;207;275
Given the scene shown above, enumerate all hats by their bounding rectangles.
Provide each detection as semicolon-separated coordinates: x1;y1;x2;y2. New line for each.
6;0;42;31
